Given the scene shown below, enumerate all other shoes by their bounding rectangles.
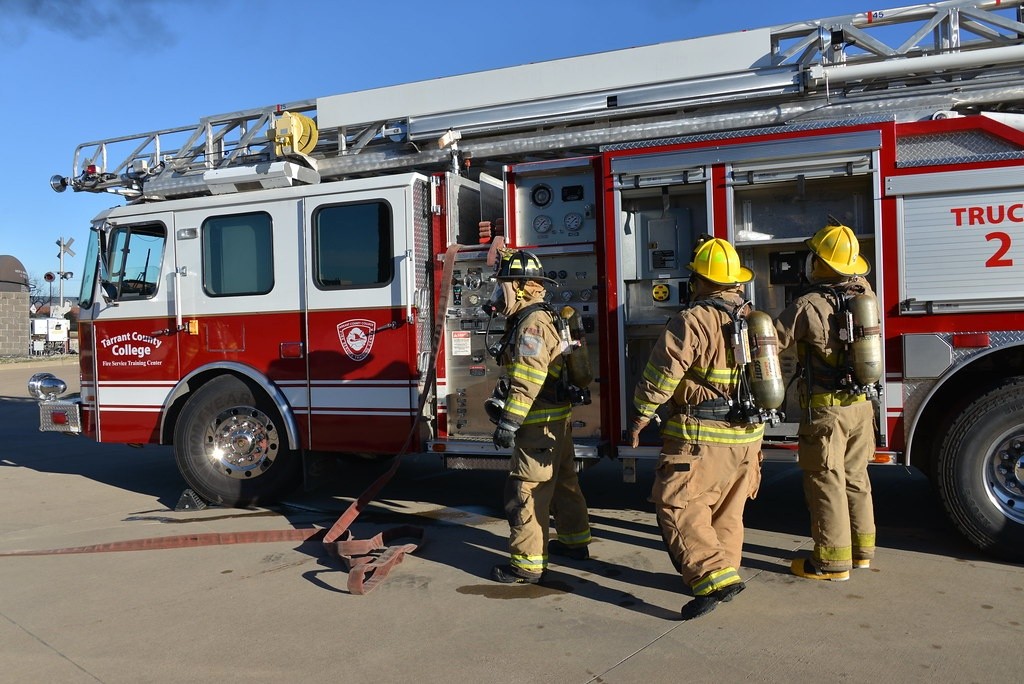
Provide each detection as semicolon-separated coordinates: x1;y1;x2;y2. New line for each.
682;580;746;619
790;556;849;583
852;553;871;570
490;562;542;586
547;540;589;560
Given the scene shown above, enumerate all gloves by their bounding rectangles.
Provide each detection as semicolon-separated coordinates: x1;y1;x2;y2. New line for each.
492;425;516;450
627;413;650;447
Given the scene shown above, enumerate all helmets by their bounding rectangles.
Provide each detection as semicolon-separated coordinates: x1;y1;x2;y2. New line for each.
685;237;756;286
804;225;871;277
494;248;556;284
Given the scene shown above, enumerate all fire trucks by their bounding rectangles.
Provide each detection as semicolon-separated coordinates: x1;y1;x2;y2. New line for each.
28;0;1024;565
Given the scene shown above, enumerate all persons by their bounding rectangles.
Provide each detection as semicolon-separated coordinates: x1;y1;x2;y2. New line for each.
772;224;877;583
481;251;590;582
631;238;766;620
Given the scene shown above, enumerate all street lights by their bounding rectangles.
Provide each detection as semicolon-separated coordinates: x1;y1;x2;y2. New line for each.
60;271;73;353
